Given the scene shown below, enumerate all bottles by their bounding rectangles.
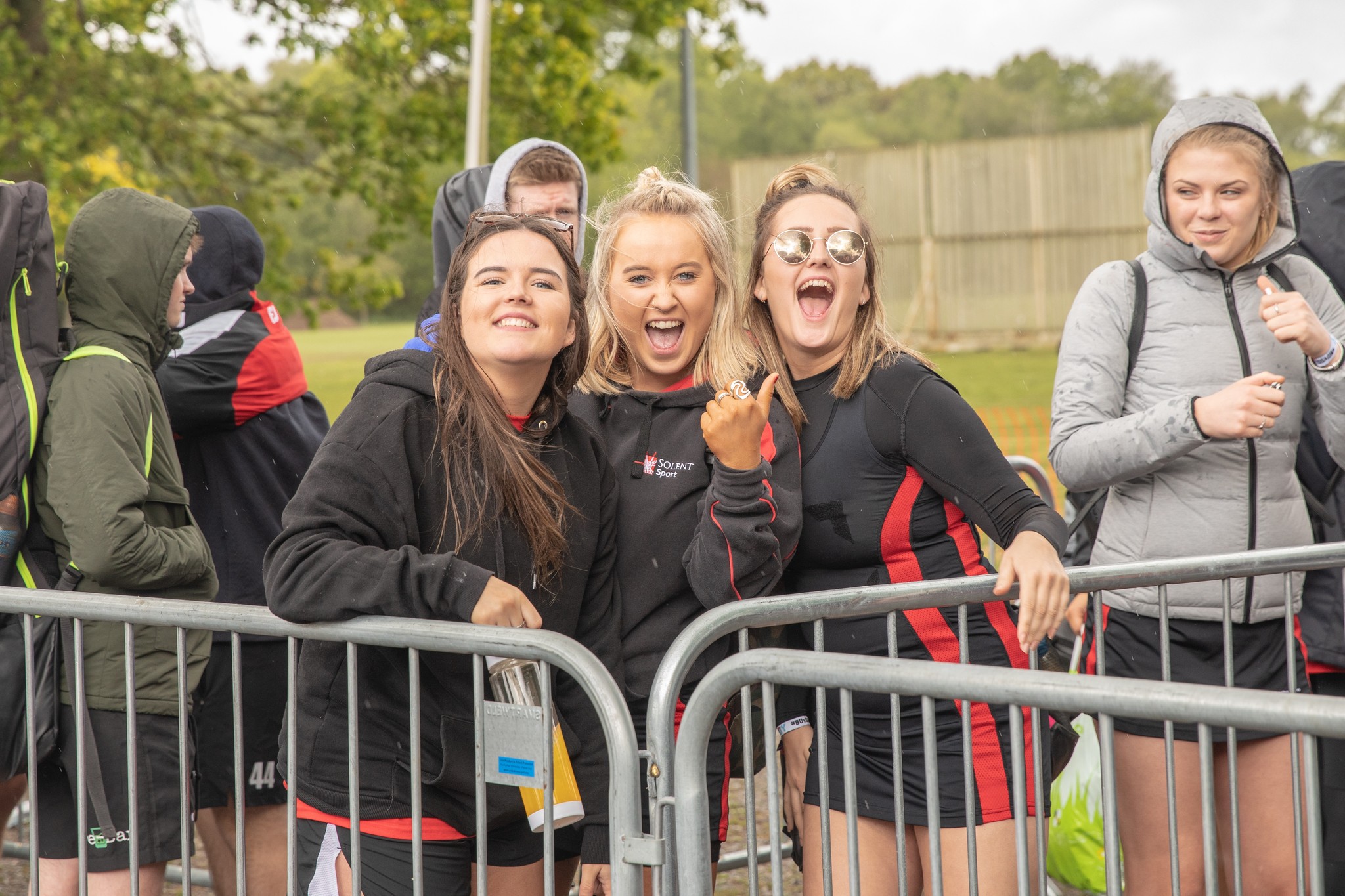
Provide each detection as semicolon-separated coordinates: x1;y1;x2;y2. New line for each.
484;654;585;834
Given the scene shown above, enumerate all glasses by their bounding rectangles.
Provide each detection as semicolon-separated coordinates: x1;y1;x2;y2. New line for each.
447;212;576;256
762;228;868;266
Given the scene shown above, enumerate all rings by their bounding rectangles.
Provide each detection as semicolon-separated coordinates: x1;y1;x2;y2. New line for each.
1274;303;1281;315
516;621;525;628
1257;414;1266;429
730;379;750;400
718;392;735;407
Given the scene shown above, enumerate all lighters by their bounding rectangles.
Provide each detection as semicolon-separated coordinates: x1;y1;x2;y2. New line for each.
1270;382;1281;390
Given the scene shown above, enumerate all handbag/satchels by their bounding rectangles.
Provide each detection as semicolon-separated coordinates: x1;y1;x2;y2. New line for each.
1046;713;1124;894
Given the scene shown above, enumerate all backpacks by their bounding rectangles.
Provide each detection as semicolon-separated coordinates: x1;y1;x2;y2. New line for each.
2;181;67;779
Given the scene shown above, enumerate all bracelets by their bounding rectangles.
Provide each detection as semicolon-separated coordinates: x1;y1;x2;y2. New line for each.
777;715;811;736
1313;334;1337;367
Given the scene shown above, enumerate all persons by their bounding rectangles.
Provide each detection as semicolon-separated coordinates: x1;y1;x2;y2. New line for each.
1049;94;1345;896
0;136;1345;896
